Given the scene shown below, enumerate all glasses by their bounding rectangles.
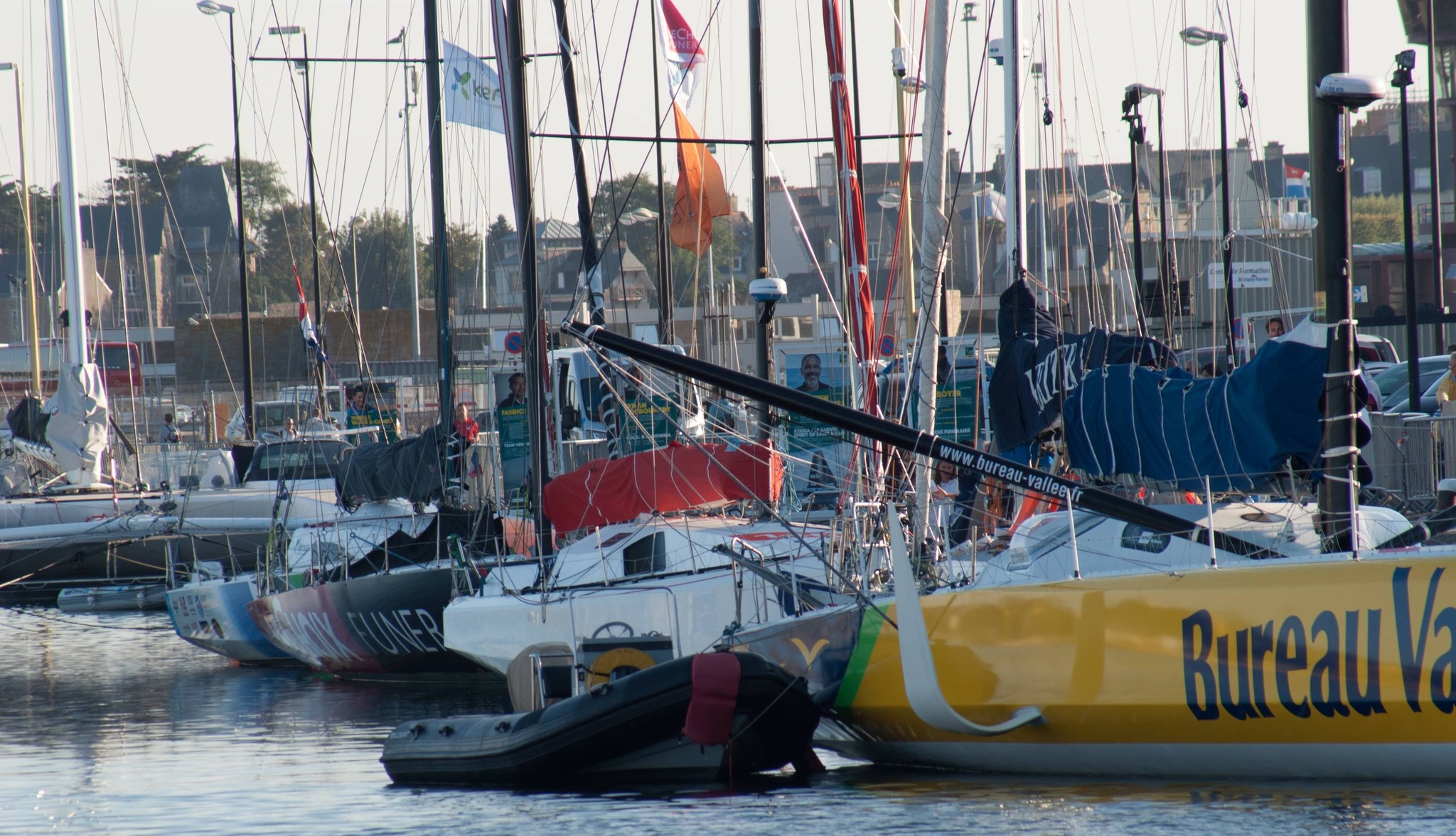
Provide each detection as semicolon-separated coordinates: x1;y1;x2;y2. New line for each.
1448;365;1455;368
1184;368;1190;372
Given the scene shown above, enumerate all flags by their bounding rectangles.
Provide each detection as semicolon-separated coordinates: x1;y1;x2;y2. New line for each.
444;39;506;134
1285;164;1311;200
660;0;705;115
294;263;329;366
669;100;731;258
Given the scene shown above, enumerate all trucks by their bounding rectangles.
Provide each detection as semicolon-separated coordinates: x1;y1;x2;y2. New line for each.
0;337;143;412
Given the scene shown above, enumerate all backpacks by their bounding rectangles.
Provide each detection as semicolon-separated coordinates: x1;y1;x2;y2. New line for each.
164;425;181;444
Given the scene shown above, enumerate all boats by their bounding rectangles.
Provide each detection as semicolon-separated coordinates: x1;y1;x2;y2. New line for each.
378;621;830;795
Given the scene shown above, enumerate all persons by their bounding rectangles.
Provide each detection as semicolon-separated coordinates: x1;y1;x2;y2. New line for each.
497;373;527;409
625;366;647;399
278;417;299;440
931;440;981;543
795;353;832;393
1436;344;1456;411
455;403;480;444
1358;359;1384;412
1183;359;1222;379
931;460;960;496
304;409;330;431
1266;318;1285;338
347;386;376;416
705;386;736;433
157;413;183;444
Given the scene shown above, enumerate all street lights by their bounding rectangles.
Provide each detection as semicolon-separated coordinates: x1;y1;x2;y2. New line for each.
1178;27;1236;375
1;61;42;400
1119;86;1146;338
267;24;328;425
1124;84;1176;351
1390;48;1419;412
195;0;255;443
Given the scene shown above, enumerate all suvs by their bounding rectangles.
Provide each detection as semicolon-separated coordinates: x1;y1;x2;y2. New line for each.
224;399;343;446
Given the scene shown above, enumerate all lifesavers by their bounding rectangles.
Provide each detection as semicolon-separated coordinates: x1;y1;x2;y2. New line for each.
587;647;657;689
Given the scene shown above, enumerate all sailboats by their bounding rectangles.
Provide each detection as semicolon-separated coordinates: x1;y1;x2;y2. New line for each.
0;0;1456;787
